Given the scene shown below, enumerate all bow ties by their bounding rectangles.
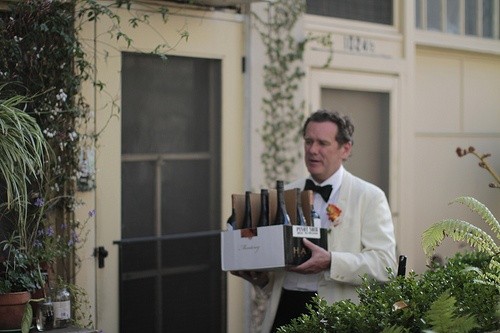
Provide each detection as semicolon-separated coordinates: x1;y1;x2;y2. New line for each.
304;179;333;203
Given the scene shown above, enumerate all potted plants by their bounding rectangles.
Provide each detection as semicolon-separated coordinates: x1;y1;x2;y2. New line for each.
0;253;36;328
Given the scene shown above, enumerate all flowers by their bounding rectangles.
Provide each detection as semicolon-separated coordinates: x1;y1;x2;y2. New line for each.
326;204;343;221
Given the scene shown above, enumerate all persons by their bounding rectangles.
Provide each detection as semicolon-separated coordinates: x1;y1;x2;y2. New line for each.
230;110;396;333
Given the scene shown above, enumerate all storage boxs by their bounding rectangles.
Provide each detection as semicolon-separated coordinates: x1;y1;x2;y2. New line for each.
221;225;328;270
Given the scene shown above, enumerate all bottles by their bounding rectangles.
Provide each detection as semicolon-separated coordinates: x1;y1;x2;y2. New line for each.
227;194;237;230
311;205;321;228
240;190;252;229
257;189;269;227
294;187;308;226
272;179;291;225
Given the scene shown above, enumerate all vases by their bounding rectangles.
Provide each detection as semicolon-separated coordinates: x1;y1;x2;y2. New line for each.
37;288;70;332
